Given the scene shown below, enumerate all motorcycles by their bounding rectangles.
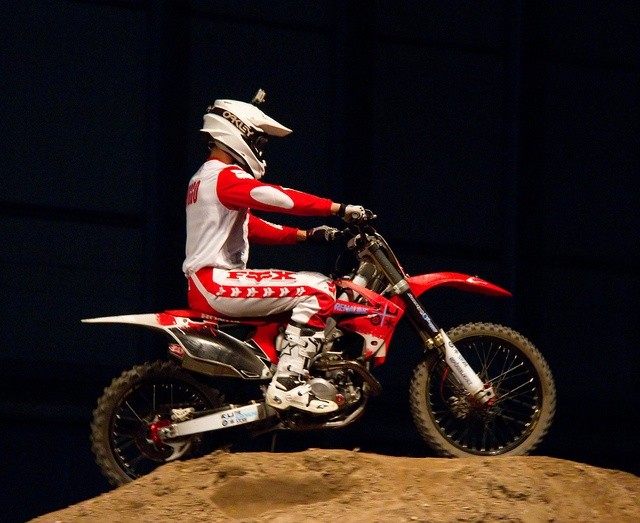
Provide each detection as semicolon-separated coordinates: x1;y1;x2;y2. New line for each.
80;197;558;489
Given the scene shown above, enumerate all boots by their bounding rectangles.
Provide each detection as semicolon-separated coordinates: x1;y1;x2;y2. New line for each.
264;318;340;415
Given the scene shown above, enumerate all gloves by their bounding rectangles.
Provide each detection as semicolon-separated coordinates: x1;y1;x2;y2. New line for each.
304;224;340;241
336;203;367;225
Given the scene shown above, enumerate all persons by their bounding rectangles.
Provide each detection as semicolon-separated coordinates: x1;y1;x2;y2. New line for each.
182;96;366;414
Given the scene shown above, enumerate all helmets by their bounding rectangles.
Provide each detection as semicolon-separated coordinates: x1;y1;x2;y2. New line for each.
200;79;294;180
